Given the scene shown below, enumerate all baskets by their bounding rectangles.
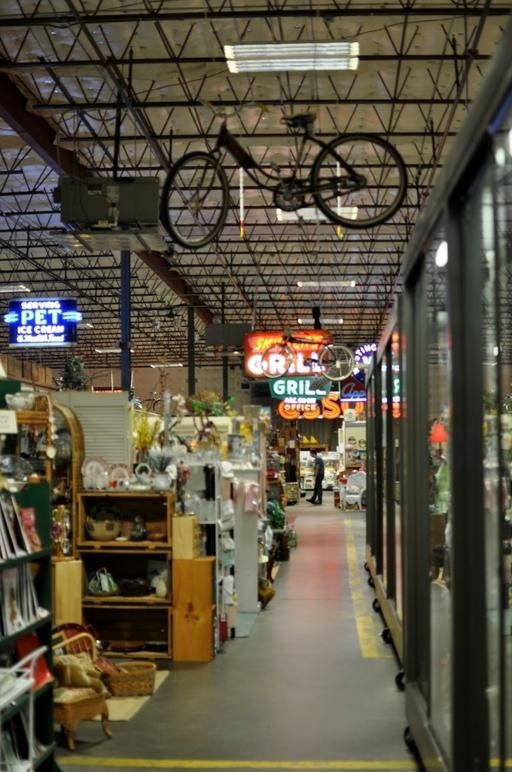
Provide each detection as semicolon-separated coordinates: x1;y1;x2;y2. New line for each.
109;663;155;698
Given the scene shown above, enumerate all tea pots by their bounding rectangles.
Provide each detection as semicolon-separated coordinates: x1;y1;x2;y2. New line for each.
4;390;39;410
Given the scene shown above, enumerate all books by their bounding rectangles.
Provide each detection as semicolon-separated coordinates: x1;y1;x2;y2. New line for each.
1;493;56;772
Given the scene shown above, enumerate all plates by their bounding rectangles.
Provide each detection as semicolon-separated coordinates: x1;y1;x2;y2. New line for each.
147;533;166;541
85;503;123;541
80;457;135;493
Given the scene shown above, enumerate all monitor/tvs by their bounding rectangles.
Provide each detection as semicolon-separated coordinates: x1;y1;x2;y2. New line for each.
6;297;79;349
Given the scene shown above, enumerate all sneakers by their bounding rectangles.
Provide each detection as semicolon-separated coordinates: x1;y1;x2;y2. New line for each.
306;499;322;505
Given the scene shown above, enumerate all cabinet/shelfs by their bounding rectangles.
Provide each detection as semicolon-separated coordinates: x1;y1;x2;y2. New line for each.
150;414;259;645
77;490;172;660
0;393;51;772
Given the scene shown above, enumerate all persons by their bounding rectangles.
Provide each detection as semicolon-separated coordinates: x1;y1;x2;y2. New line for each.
306;449;325;504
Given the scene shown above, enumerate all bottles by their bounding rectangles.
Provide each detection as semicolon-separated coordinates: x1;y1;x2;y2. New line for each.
156;576;167;598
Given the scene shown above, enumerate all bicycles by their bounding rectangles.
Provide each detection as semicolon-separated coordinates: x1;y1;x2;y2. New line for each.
158;90;410;251
261;329;355;381
141;393;163;414
